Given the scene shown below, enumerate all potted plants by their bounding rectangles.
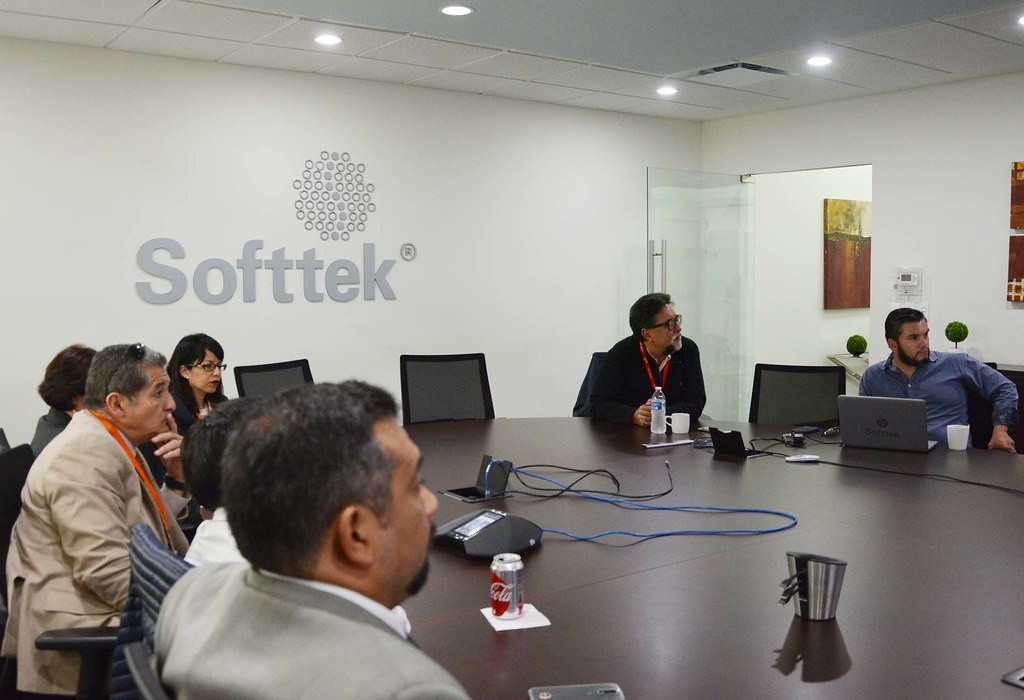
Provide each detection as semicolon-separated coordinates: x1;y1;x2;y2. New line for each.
945;321;968;353
846;335;867;358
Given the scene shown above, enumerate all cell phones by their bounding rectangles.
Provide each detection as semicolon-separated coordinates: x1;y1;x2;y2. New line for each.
446;511;503;542
792;426;820;433
529;683;625;700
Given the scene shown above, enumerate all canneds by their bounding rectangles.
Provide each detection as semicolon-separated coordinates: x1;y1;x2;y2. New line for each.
490;553;524;620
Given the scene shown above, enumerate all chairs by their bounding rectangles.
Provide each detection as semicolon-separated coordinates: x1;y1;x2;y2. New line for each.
400;353;494;426
967;363;1024;454
0;429;119;700
749;364;846;430
234;359;314;398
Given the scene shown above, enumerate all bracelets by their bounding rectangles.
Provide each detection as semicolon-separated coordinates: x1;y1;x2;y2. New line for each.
164;475;186;490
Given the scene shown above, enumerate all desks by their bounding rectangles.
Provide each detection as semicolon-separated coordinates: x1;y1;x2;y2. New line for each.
399;417;1023;700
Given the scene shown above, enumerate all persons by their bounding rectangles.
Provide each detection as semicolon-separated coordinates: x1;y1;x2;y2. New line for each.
859;308;1018;455
0;342;192;696
180;397;263;566
165;333;230;433
31;344;156;476
588;293;706;428
152;380;471;700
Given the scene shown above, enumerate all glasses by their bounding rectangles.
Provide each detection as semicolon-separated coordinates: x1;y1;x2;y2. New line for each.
105;343;145;397
184;362;227;372
640;315;682;331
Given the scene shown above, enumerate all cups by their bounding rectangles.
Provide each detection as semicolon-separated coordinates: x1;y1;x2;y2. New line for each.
665;413;690;434
947;425;969;450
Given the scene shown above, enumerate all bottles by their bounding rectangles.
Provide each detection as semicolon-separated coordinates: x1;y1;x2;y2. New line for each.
650;386;666;434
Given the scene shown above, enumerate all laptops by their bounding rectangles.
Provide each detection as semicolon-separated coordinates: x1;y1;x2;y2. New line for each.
838;394;939;451
